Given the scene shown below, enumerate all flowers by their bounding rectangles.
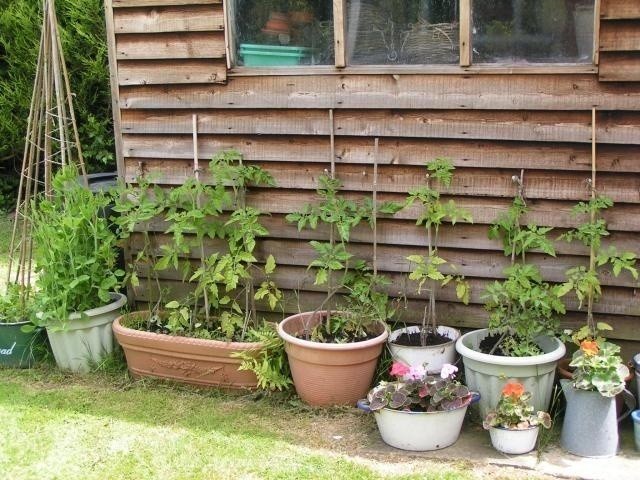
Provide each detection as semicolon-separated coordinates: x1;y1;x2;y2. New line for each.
483;379;552;432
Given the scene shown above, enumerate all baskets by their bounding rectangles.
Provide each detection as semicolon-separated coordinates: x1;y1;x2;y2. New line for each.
402;22;460;64
319;1;399;63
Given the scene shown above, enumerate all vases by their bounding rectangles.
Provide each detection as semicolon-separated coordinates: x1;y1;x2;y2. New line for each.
357;381;481;453
559;378;619;459
489;426;539;455
632;352;640;453
